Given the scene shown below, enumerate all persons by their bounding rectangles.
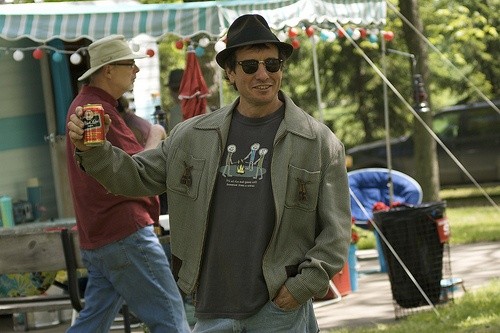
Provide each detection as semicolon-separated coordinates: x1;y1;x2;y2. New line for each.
66;14;351;333
60;34;189;332
161;69;186;136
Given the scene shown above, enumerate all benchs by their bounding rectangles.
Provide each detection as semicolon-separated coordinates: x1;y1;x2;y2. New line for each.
0;295;132;333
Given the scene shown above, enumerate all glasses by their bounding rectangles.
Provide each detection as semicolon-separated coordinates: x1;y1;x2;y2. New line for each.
105;61;137;69
235;57;284;74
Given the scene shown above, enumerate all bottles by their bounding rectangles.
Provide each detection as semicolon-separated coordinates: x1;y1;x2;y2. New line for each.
0;196;16;228
152;106;166;129
13;312;26;333
27;178;43;221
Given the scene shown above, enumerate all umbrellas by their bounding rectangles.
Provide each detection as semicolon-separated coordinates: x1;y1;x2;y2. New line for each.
177;44;210;118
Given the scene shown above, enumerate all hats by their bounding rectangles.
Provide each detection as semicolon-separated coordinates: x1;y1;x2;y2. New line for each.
78;34;150;81
215;14;294;68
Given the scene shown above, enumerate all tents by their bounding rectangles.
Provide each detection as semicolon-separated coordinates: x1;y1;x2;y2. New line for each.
1;0;394;217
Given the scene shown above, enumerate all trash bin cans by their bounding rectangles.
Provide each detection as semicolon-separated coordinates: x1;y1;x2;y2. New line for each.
374;201;450;308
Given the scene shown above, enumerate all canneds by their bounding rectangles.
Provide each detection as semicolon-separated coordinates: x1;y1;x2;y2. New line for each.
82;104;105;147
13;312;26;332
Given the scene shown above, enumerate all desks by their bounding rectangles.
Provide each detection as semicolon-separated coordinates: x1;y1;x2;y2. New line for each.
0;214;170;333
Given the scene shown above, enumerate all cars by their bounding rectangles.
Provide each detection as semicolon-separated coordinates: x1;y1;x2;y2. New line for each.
345;100;500;192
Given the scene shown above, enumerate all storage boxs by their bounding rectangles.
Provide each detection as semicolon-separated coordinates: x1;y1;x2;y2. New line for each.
26;285;73;329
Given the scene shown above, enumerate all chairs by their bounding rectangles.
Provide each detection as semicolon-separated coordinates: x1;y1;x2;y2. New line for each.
348;167;423;291
